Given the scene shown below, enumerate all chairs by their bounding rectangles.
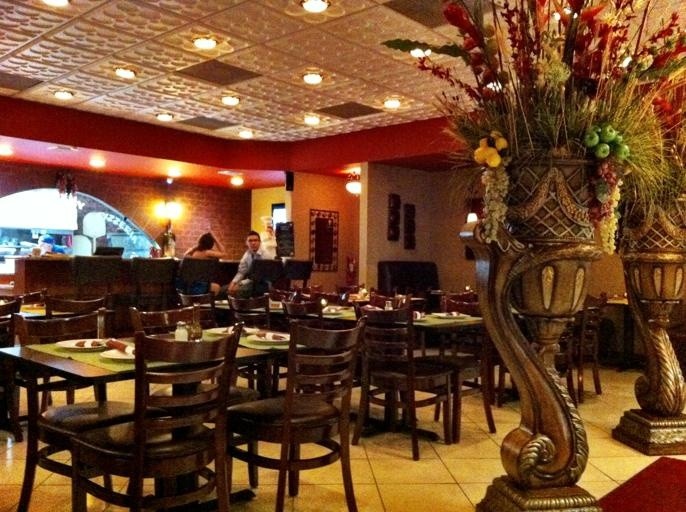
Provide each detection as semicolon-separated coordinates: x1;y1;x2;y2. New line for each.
0;256;607;512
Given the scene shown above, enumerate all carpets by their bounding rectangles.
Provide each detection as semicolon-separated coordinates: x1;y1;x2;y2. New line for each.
596;457;686;511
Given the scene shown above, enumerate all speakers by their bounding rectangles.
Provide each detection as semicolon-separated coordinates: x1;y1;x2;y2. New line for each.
285;171;294;191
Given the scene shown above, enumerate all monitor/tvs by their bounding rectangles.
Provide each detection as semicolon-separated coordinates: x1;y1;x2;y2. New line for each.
95;246;124;255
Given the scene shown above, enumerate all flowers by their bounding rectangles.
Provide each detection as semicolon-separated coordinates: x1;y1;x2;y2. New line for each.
380;0;686;260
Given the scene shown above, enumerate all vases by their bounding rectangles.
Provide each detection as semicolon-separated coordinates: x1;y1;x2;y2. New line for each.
472;158;606;512
612;193;686;456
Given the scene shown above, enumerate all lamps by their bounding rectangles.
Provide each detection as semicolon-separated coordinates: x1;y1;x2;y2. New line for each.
346;171;362;197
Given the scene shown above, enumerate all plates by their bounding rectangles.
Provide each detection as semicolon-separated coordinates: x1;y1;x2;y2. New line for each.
53;338;114;352
433;311;470;320
246;333;291;344
98;347;137;361
204;325;260;336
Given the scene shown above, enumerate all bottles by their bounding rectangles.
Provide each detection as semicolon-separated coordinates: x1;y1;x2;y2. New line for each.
385;301;393;311
175;321;188;342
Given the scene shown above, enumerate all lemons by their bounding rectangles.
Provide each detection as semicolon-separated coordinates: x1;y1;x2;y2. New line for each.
474;131;509;168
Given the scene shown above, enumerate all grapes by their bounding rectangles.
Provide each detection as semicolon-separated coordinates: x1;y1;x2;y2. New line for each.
589;159;624;256
480;149;513;245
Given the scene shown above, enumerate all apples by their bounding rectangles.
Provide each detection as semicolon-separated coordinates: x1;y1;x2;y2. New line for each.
584;123;629;161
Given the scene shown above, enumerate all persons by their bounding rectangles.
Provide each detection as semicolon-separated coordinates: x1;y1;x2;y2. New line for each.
218;230;272;300
36;234;72;257
174;227;229;299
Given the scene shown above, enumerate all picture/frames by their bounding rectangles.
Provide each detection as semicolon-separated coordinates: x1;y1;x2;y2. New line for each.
310;209;338;272
